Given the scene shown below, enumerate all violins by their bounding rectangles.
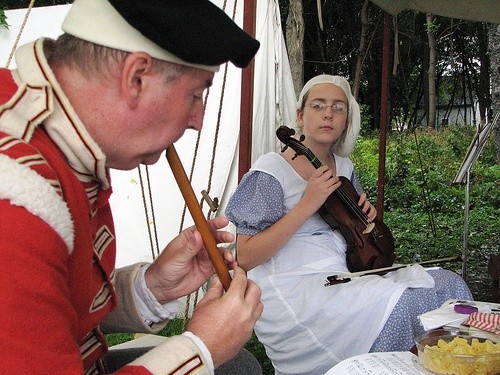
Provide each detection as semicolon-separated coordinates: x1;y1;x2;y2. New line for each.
275;126;394;277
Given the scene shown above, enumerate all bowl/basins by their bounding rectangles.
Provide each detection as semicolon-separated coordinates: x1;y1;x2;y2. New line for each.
414;328;500;375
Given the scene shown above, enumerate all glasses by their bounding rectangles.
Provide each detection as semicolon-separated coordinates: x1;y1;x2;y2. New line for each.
303;100;349;114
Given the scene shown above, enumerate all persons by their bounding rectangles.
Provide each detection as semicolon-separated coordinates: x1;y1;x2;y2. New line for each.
225;73;475;375
1;0;264;375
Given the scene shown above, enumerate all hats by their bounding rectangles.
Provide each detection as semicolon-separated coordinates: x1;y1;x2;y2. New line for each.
60;0;260;73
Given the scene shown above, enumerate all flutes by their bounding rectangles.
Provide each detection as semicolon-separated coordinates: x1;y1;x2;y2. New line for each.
166;142;232;293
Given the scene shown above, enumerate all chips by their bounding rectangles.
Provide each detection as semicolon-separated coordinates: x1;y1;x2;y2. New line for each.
423;336;500;375
